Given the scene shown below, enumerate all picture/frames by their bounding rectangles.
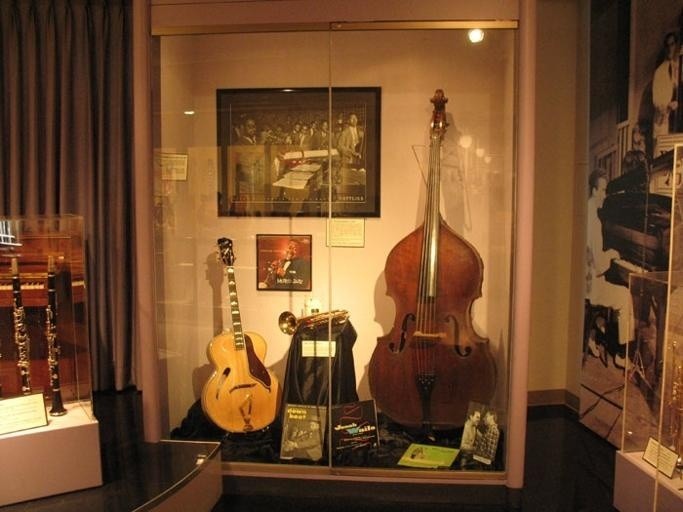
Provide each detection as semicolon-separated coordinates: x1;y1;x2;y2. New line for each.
213;85;382;219
256;233;313;292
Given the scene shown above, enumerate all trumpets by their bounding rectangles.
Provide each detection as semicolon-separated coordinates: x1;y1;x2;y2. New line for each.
279;310;350;335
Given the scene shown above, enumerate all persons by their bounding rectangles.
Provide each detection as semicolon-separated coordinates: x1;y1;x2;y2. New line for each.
477;410;500;462
460;410;481;454
650;31;681;161
283;414;323;461
226;111;365;216
270;238;310;290
586;166;636;370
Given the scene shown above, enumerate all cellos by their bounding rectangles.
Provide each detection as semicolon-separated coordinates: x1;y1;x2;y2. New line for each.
368;89;498;428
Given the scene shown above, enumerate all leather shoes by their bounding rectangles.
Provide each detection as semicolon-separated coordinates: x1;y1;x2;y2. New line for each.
615;356;633;369
588;336;600;357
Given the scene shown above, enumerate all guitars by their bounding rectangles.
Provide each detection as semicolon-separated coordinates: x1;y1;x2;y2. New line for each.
202;238;282;433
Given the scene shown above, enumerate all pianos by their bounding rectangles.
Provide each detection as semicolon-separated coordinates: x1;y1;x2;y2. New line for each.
599;149;672;379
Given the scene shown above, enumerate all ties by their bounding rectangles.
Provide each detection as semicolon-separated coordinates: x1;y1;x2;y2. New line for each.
668;64;673;79
